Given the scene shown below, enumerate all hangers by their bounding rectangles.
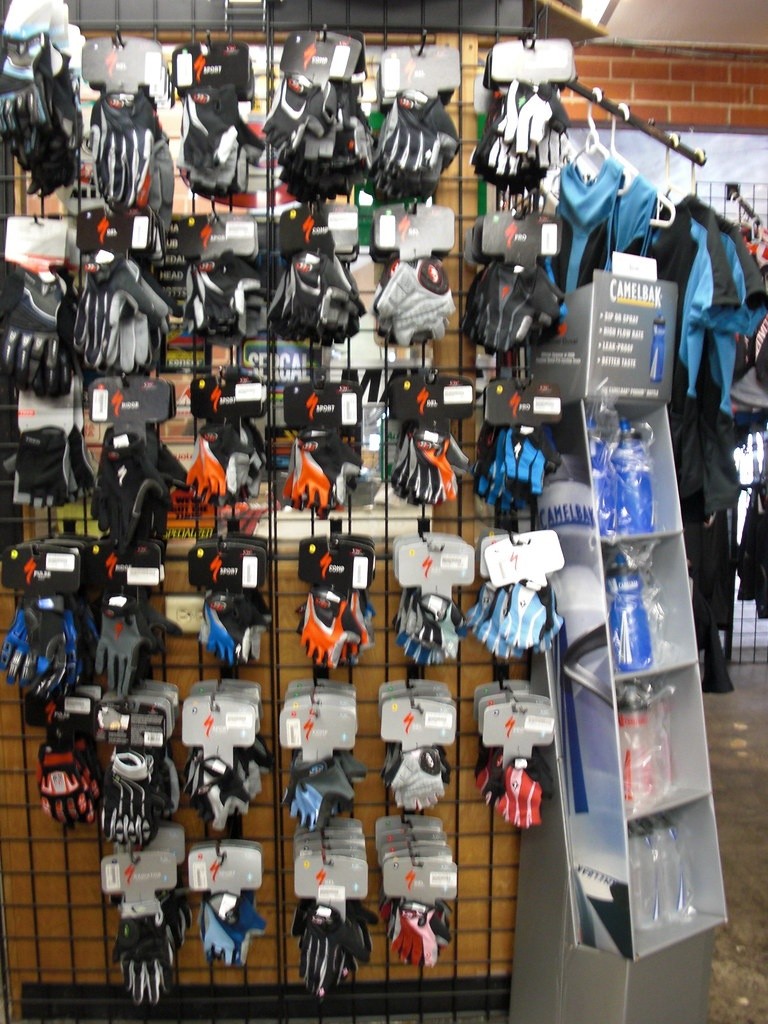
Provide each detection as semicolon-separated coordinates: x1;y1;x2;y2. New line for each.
548;86;763;247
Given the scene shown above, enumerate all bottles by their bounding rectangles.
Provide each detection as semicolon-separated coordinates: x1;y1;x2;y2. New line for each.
650;814;687;918
648;310;666;381
612;418;655;533
605;554;653;670
585;420;613;534
618;680;673;803
628;816;660;931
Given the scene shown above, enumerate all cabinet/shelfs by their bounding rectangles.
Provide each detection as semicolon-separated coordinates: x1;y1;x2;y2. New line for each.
507;272;730;1023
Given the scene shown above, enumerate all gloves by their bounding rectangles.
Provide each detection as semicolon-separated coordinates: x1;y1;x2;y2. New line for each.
0;0;571;1007
